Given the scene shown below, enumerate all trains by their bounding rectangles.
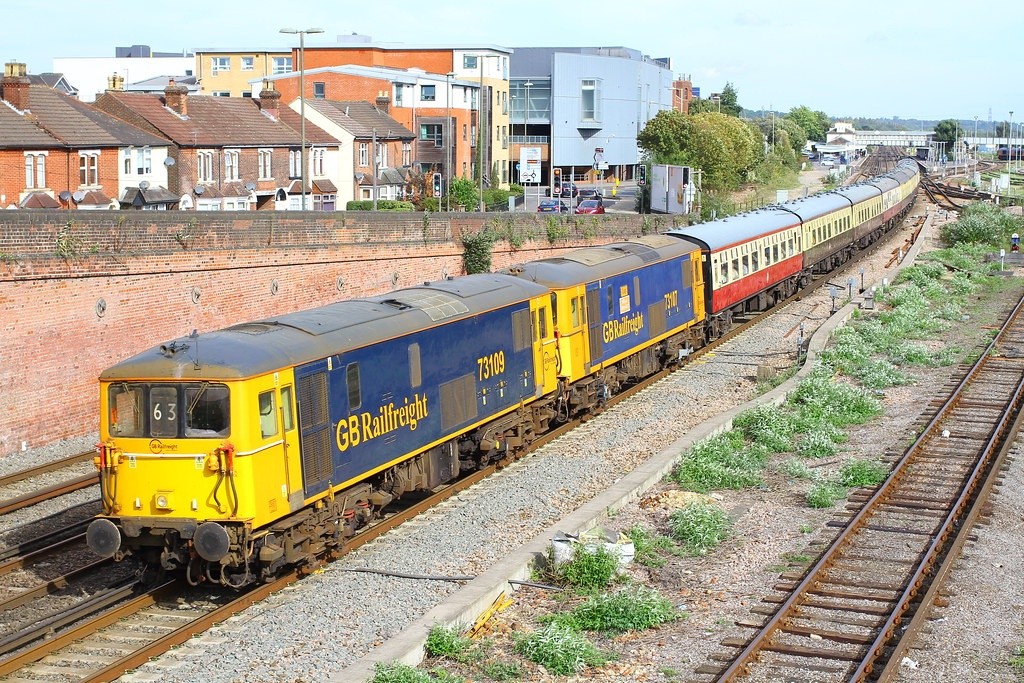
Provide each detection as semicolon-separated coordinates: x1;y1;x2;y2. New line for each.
85;156;924;591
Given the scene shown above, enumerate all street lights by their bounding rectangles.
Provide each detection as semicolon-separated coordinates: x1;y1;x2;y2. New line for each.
666;87;685;115
279;27;326;212
711;96;721;114
524;80;533;145
1007;111;1016;195
447;72;458;214
973;116;980;182
770;111;776;154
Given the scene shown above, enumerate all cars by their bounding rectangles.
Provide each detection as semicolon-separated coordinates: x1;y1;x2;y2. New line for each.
820;158;835;166
576;188;604;206
544;181;578;198
537;199;568;213
575;199;606;214
801;152;815;159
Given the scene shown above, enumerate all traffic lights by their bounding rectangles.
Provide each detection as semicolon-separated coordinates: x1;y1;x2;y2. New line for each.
639;164;646;186
552;168;562;196
432;172;442;199
